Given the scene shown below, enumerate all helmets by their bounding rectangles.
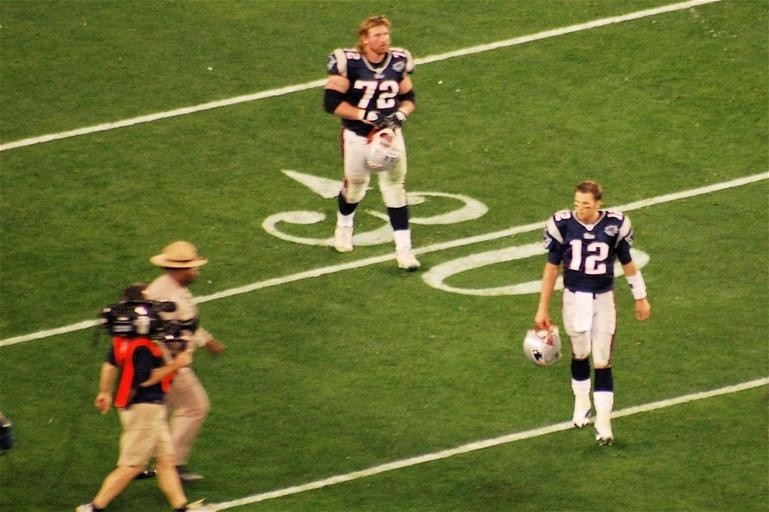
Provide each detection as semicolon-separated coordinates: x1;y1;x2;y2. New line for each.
522;325;564;367
366;131;404;168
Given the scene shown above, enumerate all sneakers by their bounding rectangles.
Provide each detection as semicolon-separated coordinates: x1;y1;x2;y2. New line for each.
592;422;616;445
572;404;593;428
75;503;94;512
135;464;204;511
395;250;422;270
332;224;358;252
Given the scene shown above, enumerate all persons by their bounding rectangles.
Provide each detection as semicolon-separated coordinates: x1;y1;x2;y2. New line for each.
534;182;651;447
76;285;218;512
134;241;225;480
323;14;422;269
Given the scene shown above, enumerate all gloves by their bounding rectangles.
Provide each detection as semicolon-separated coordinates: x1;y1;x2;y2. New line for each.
386;111;407;131
364;110;385;130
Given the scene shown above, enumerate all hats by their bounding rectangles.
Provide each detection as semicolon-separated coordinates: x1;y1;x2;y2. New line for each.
148;241;208;269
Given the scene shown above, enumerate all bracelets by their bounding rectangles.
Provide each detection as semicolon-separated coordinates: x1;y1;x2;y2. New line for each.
625;270;647;299
